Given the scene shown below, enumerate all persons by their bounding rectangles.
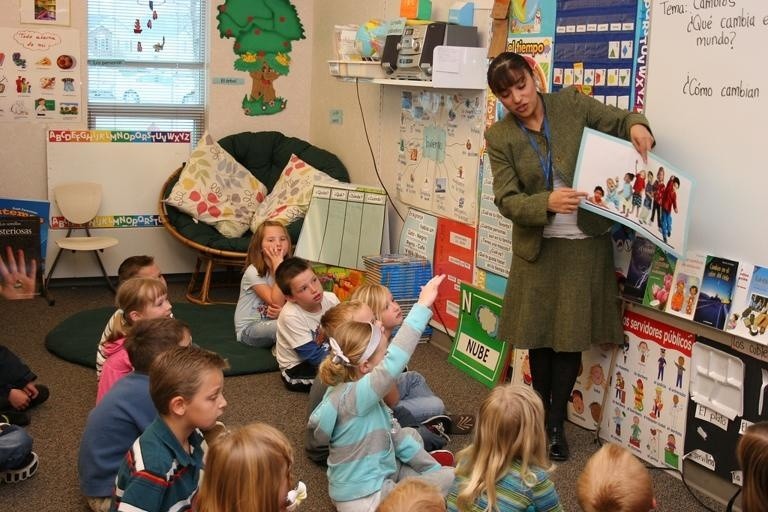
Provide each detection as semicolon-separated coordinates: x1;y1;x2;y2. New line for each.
190;421;308;512
483;53;657;461
77;317;195;512
112;345;227;512
0;245;38;299
573;443;657;512
569;279;741;455
522;355;532;385
585;159;680;244
739;424;768;512
96;278;174;404
0;342;50;429
96;255;168;387
446;383;566;512
36;98;46;113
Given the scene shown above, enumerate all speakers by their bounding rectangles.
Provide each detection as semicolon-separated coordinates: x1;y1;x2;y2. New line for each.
420;23;478;76
381;35;401;74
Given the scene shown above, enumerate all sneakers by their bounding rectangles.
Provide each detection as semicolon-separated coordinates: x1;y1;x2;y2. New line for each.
0;409;29;433
421;415;450;439
0;451;39;483
429;449;454;467
28;384;50;409
451;414;474;434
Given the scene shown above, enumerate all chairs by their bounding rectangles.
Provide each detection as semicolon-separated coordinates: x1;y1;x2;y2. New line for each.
155;130;351;307
37;181;118;308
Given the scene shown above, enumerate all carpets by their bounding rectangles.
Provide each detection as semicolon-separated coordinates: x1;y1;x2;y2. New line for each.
44;303;283;377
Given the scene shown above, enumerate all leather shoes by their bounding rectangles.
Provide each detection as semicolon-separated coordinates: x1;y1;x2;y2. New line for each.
547;426;569;460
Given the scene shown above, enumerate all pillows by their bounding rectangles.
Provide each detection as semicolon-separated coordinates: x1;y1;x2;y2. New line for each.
249;153;339;234
158;130;268;239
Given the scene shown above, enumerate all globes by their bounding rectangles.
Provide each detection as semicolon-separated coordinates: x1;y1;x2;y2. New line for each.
354;19;387;61
